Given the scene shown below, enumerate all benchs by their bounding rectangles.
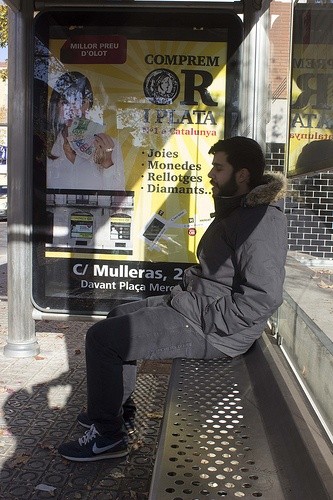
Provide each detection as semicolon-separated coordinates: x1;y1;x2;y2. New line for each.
147;324;333;500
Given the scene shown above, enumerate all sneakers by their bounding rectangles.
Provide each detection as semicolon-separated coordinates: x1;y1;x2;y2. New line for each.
57;425;130;462
78;409;136;433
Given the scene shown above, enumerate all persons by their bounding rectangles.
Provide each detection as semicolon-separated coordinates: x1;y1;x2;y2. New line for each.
46;70;126;190
54;134;291;464
